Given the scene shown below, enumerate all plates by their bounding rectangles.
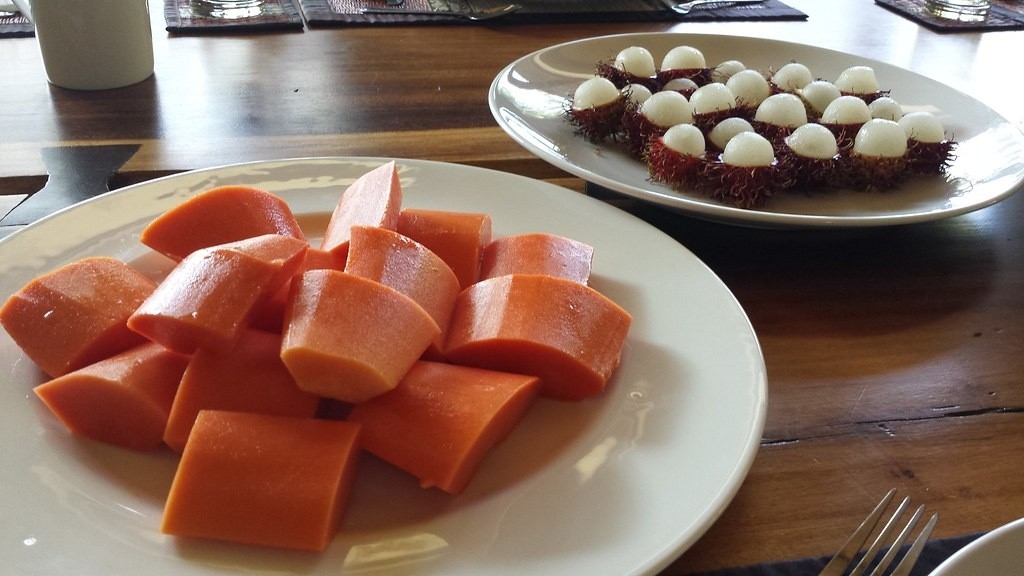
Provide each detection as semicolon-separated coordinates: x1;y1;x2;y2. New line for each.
487;30;1024;227
925;517;1024;576
0;154;770;576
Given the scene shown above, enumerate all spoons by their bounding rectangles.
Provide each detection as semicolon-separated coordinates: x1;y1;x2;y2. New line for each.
659;0;763;15
361;4;523;20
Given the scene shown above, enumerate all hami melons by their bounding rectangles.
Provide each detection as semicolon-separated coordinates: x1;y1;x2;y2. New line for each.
1;158;633;553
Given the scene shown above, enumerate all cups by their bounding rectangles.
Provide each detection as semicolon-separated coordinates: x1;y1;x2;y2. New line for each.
29;0;156;91
190;0;265;19
923;0;992;28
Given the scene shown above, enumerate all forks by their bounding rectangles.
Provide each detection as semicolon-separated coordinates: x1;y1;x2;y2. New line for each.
817;487;940;576
